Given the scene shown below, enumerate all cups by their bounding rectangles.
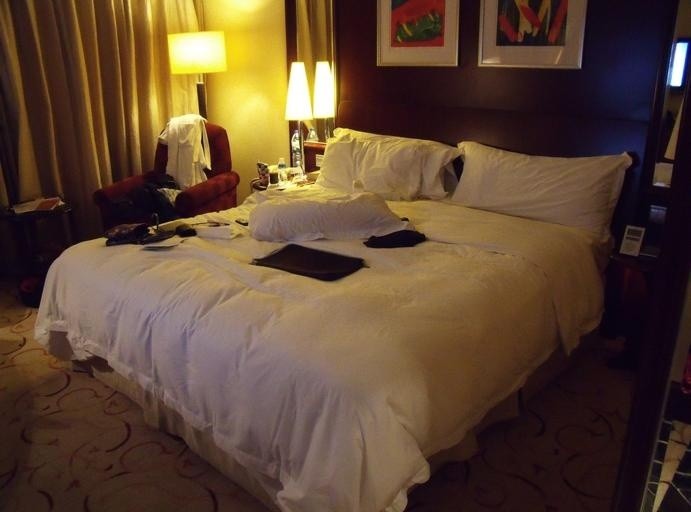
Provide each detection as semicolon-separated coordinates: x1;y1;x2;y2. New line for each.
268;165;279;184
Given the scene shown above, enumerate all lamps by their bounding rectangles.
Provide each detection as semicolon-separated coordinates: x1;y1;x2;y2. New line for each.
283;58;312;167
313;61;335;119
167;30;227;118
665;38;688;88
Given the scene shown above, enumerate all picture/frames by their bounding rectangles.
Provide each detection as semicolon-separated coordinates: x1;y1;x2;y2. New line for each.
376;0;588;70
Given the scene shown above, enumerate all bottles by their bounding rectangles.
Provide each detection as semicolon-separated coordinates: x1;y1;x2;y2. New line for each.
278;158;286;181
290;129;303;167
294;159;303;177
306;128;319;143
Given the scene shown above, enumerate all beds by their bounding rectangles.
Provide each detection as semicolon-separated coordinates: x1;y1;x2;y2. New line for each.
35;119;647;512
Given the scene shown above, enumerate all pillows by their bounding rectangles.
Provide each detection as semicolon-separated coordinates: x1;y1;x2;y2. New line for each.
332;128;462;200
249;191;416;242
445;141;630;234
316;136;433;200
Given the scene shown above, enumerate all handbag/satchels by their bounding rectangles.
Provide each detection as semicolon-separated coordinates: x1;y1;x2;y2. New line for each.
104;223;150;239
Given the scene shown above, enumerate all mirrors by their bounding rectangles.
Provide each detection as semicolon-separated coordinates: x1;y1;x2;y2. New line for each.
287;1;337;168
633;0;690;187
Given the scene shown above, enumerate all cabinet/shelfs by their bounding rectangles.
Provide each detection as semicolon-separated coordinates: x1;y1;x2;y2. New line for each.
0;195;75;281
637;277;691;512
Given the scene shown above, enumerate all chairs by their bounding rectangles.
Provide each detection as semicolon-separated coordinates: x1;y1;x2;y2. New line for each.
92;120;239;218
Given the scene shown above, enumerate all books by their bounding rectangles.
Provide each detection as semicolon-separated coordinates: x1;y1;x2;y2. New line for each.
35;198;60;211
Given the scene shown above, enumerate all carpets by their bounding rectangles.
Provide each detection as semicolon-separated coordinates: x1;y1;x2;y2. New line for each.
0;280;659;512
